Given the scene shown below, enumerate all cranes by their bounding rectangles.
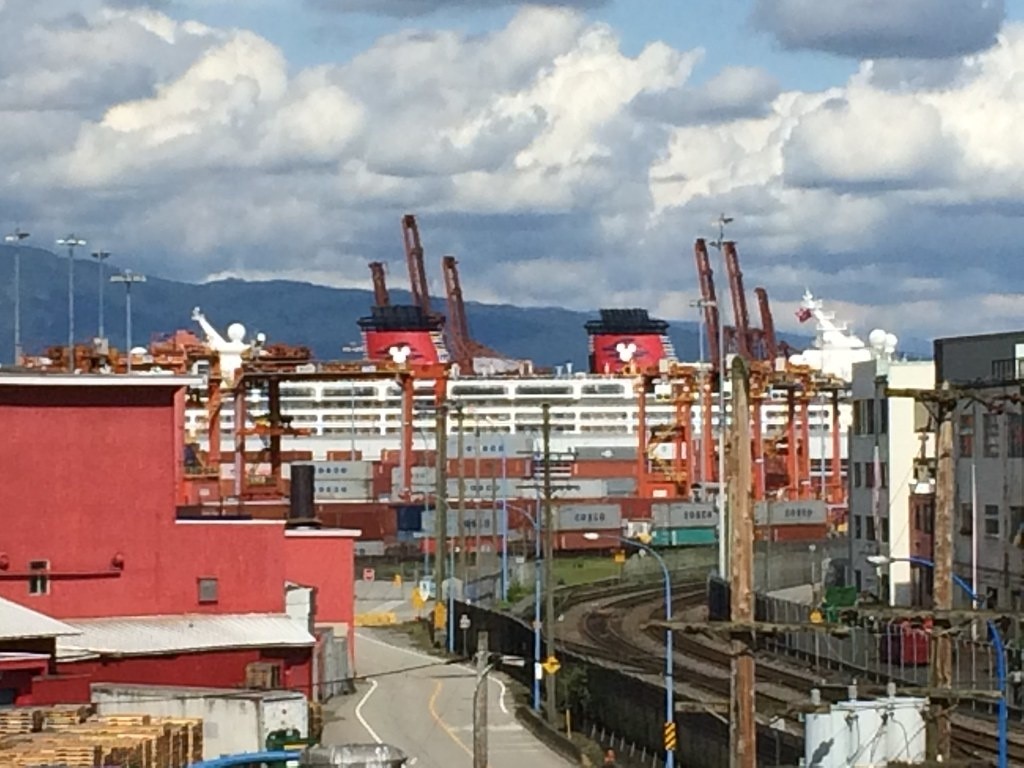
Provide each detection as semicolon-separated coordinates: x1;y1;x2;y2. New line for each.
692;236;725;375
754;286;778;363
367;261;390;308
721;240;754;359
443;255;475;375
401;213;430;311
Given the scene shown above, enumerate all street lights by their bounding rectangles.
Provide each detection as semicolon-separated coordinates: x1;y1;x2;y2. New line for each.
55;232;88;374
866;553;1009;768
580;531;677;768
2;228;32;367
190;743;407;768
